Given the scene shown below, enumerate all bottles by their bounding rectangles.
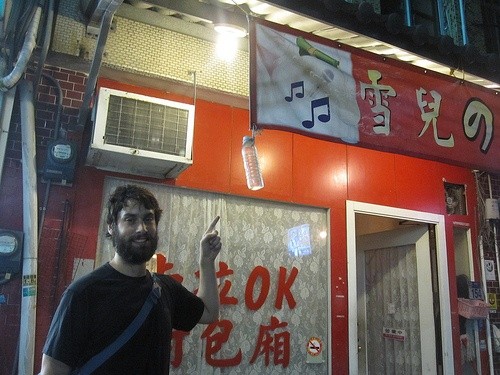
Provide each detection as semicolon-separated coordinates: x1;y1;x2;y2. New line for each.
241;136;264;191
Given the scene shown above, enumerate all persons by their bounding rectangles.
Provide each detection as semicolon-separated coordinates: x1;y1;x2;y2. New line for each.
37;184;223;375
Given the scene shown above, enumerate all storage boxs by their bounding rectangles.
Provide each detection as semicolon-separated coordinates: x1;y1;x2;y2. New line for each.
458;298;492;321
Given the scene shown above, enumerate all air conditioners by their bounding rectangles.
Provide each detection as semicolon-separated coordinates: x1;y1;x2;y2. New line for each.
79;86;195;184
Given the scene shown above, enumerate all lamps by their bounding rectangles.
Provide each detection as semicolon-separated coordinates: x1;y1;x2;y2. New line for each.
210;20;249;40
